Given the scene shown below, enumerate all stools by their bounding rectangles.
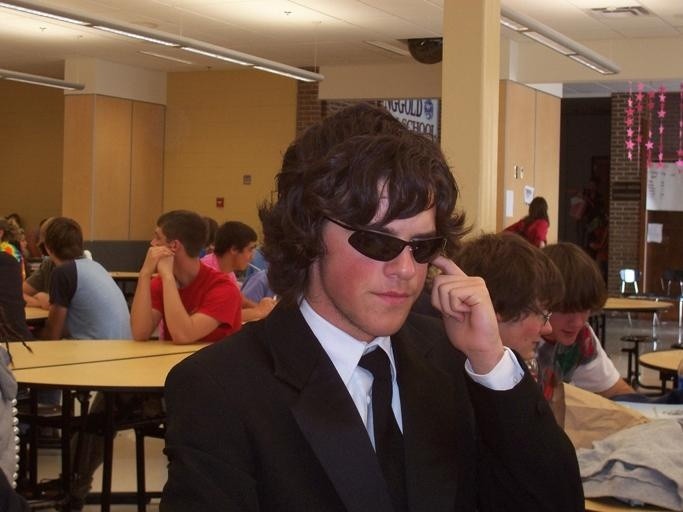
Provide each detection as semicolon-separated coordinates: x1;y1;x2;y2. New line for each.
619;334;659;384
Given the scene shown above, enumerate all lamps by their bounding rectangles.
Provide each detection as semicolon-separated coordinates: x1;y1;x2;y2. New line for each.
568;54;615;75
522;30;578;56
93;19;182;49
501;14;530;32
0;1;93;27
251;54;324;83
179;33;255;67
0;68;86;92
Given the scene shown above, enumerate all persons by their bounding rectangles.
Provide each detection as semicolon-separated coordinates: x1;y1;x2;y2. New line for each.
38;216;132;439
4;213;28;257
240;269;276;304
159;102;585;512
539;243;635;398
130;210;241;345
200;221;276;323
453;231;566;430
0;220;26;281
0;251;36;342
23;223;86;311
504;196;550;248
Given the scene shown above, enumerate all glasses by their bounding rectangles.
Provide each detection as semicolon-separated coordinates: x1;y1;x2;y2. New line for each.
532;307;553;327
319;212;447;264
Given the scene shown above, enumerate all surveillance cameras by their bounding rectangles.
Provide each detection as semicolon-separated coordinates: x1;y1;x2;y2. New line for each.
407;37;443;65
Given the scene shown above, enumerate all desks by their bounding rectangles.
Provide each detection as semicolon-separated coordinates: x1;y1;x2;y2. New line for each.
603;296;673;312
0;339;214;512
24;307;50;321
637;347;683;394
108;270;140;295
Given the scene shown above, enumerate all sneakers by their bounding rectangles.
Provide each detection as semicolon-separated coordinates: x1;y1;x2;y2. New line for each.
55;494;83;511
33;479;70;498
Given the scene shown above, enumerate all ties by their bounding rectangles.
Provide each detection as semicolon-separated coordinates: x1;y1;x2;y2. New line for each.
359;350;405;472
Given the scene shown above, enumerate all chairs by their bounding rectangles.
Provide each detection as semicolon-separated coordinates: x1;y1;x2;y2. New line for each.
618;268;643;326
652;269;683;328
